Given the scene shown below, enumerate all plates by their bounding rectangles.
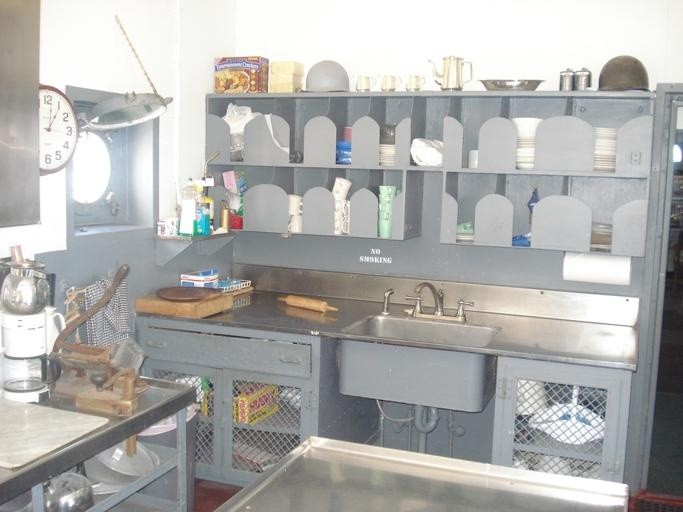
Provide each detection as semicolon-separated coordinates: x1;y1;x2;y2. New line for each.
477;79;546;91
379;143;396;167
592;127;618;172
512;117;545;170
156;286;221;303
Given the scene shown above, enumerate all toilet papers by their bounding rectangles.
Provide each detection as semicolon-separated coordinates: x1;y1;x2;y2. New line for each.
562;253;633;287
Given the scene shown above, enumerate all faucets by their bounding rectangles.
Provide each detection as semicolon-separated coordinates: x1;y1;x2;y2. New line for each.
383;290;395;314
415;281;445;316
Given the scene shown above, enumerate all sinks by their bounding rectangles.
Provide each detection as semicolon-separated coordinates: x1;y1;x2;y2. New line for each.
337;314;500;414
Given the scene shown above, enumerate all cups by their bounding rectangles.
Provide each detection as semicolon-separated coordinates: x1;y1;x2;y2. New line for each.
355;75;376;93
381;75;402;93
378;183;397;239
331;177;352;237
405;75;425;91
287;194;304;234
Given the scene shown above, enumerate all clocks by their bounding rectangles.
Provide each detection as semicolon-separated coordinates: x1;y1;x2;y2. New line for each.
36;85;80;175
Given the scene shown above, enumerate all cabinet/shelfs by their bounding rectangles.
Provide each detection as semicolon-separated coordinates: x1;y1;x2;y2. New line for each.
132;317;342;492
488;356;633;487
204;90;668;258
1;406;198;512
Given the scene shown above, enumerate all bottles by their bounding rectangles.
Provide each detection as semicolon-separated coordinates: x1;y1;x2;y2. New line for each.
559;66;593;90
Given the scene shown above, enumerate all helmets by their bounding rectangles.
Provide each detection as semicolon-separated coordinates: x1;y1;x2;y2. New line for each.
300;59;349;92
599;55;649;90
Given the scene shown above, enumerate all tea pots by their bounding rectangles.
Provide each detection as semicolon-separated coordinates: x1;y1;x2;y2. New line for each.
428;54;473;91
